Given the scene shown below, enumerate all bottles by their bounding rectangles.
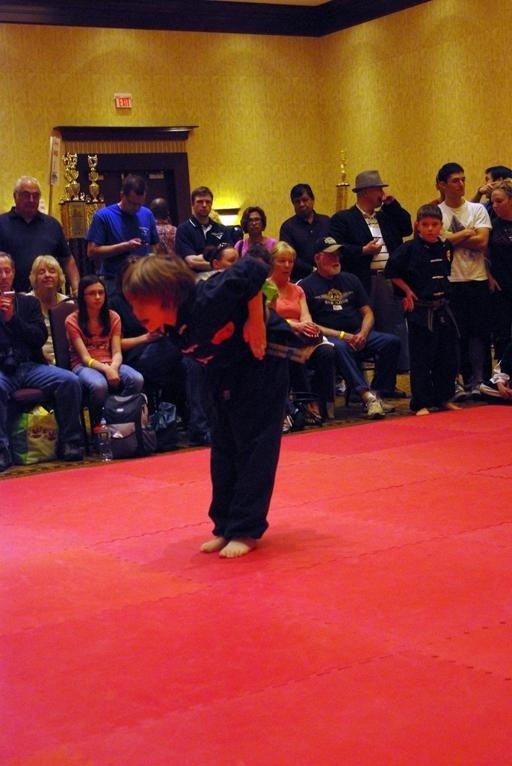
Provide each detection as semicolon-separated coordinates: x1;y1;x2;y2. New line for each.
97;418;113;463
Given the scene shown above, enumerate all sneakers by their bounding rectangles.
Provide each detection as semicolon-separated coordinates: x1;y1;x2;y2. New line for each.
0;446;14;472
358;399;396;416
449;379;485;403
366;394;387;420
188;430;214;445
334;377;347;396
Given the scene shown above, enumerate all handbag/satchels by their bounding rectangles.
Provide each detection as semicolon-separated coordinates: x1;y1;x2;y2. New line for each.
147;401;180;454
8;405;59;465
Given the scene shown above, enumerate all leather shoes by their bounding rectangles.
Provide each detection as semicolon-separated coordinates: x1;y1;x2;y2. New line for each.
59;439;84;462
370;375;407;398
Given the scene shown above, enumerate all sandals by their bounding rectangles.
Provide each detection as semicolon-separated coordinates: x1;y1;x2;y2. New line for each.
303;403;323;425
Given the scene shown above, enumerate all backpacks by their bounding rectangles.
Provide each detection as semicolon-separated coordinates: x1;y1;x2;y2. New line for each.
101;383;158;460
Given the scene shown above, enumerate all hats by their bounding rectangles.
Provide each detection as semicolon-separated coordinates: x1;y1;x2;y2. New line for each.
311;235;345;255
351;170;389;193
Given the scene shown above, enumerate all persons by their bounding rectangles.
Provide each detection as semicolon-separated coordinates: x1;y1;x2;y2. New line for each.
120;242;303;559
0;159;511;474
